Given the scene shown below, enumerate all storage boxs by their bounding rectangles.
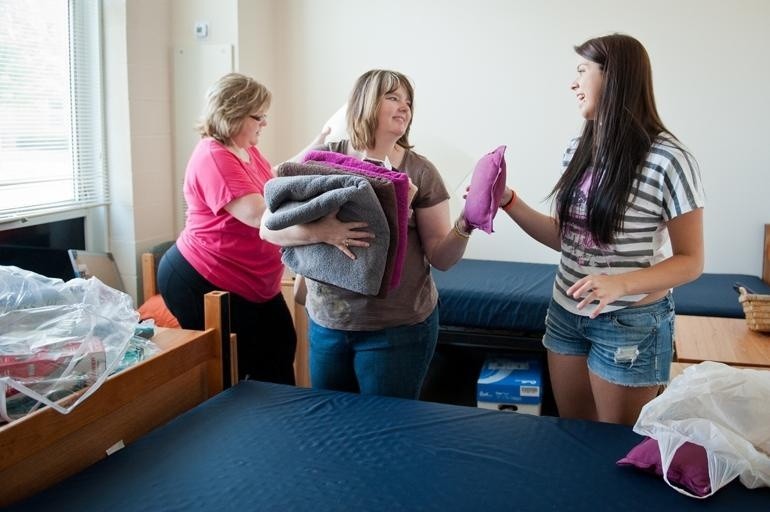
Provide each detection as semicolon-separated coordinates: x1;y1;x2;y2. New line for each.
476;354;545;417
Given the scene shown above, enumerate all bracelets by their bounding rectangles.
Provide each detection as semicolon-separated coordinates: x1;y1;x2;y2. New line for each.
501;191;517;211
453;221;469;240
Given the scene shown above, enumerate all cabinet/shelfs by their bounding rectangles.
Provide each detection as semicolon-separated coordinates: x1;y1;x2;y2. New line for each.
131;324;205;353
668;313;770;388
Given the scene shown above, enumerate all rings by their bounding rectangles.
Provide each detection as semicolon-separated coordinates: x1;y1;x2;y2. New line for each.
346;239;349;246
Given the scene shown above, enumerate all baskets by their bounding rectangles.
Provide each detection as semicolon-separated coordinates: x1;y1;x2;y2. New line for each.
738;286;770;333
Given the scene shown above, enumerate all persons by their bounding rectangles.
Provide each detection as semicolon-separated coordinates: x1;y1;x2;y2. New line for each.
259;70;476;401
154;72;296;388
463;34;705;428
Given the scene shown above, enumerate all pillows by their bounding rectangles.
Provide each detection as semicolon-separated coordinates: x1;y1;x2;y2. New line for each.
464;145;510;235
617;428;711;497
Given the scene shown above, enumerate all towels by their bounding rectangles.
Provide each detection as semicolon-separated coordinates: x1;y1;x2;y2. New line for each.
262;149;414;297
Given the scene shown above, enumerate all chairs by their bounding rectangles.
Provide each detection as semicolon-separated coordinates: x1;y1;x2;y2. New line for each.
141;239;180;300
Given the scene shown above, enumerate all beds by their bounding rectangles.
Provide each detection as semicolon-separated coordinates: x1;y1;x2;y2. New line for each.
292;219;770;389
1;287;770;512
